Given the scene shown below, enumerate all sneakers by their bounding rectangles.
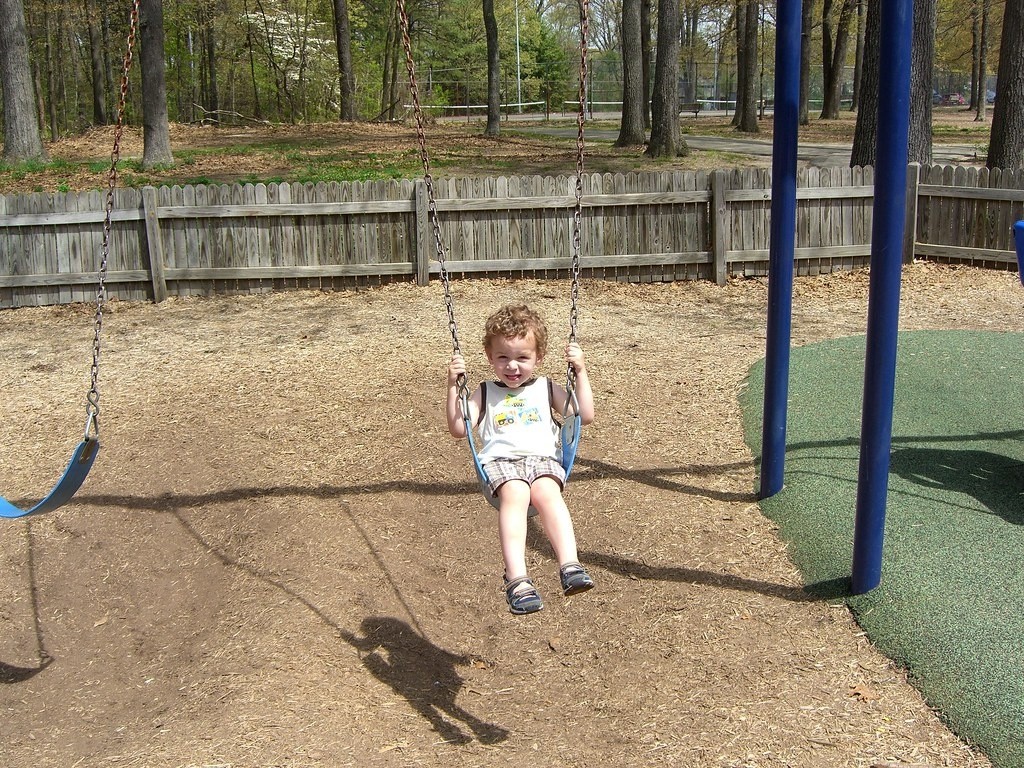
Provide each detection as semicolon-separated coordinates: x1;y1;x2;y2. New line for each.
503;569;544;615
560;565;594;596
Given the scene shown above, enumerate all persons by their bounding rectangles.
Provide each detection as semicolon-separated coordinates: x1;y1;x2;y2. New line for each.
446;305;594;615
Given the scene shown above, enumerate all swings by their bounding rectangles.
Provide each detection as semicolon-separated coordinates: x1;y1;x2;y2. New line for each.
396;1;590;517
0;0;140;518
